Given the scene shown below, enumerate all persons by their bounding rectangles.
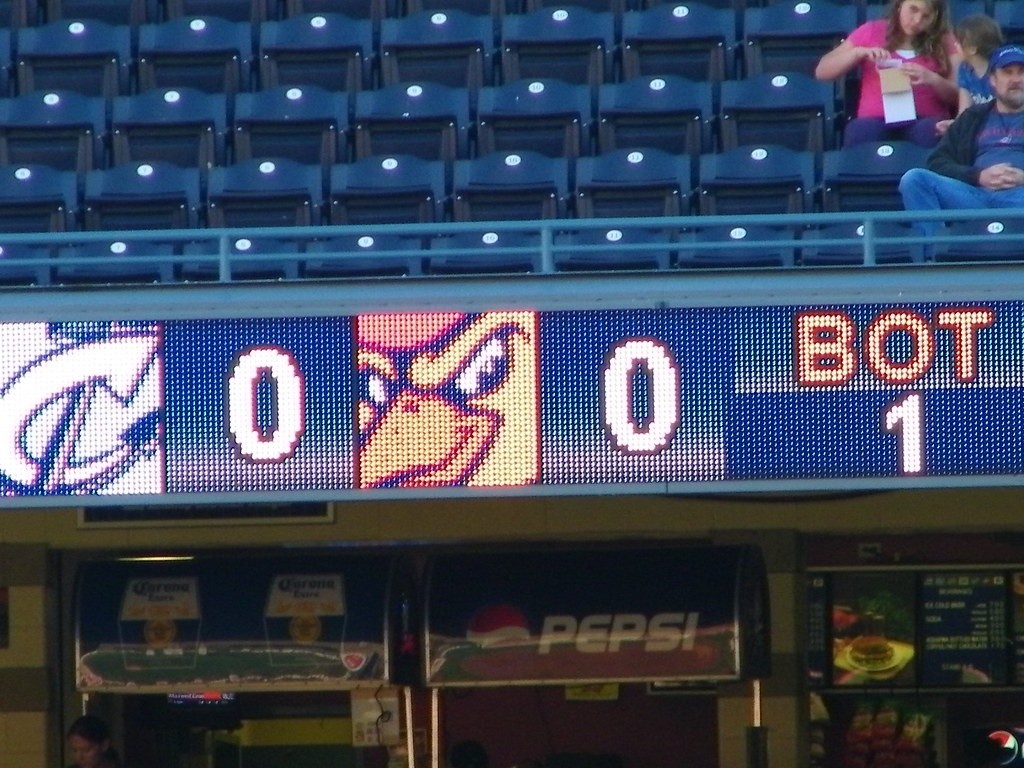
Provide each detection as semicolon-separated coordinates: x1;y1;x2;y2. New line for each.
65;715;122;768
814;1;1024;257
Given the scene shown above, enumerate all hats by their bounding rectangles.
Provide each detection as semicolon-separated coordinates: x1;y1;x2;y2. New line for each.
989;45;1024;72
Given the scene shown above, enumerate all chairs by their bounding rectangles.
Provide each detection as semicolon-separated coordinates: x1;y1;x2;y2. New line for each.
0;0;1024;283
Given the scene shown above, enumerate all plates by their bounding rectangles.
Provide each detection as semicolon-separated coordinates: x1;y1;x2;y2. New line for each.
144;618;175;646
289;613;321;644
847;643;903;672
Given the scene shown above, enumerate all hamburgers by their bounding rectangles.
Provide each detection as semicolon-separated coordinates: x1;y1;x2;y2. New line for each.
849;635;894;670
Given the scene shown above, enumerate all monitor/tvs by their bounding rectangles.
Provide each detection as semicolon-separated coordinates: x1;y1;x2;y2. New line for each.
167;692;239;712
